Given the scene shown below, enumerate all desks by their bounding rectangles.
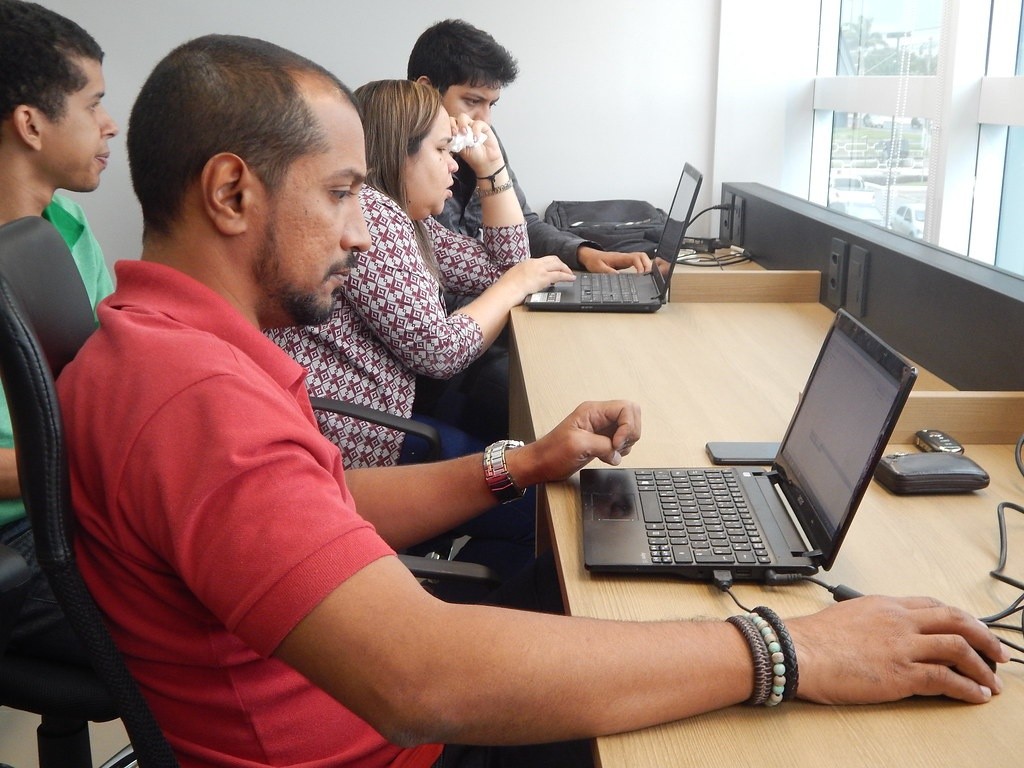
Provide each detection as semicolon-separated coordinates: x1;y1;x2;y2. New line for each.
507;238;1024;768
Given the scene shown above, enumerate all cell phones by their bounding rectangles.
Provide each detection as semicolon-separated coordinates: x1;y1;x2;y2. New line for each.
706;442;780;465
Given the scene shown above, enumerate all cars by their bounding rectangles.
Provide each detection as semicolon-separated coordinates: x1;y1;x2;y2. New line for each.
830;135;928;240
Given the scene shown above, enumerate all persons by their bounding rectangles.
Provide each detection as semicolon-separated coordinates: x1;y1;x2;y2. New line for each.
261;79;561;615
408;18;653;392
54;34;974;768
0;1;118;666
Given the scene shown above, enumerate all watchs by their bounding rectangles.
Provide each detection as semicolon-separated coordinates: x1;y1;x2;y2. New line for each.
483;439;529;505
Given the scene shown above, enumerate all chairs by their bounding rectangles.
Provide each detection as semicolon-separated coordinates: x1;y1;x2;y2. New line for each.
0;541;136;768
0;216;503;768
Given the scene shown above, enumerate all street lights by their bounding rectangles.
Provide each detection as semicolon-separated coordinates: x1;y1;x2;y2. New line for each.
888;31;911;67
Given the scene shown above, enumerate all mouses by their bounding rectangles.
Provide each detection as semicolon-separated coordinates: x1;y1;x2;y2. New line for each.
947;650;996;679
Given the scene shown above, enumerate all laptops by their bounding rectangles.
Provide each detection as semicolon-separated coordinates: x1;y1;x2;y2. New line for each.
580;307;919;580
524;161;703;312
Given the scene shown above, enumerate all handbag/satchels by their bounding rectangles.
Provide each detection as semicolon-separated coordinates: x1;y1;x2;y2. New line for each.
543;200;670;260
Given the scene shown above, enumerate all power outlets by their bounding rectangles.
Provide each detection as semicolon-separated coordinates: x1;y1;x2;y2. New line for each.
827;238;848;307
721;190;735;240
733;195;744;247
843;244;871;318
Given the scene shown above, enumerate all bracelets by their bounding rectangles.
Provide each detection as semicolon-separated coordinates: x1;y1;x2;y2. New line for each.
476;162;514;199
725;606;799;707
